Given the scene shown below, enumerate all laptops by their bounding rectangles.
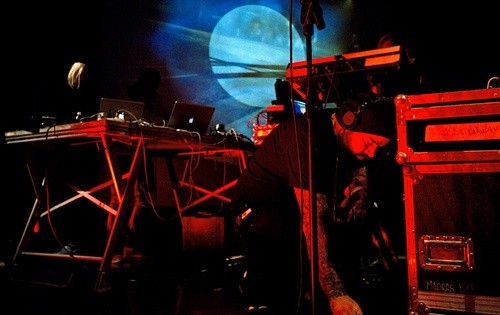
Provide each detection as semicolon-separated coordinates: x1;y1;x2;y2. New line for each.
167;101;215;134
98;97;145;121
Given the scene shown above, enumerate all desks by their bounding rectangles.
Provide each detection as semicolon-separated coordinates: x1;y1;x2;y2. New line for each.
5;120;258;292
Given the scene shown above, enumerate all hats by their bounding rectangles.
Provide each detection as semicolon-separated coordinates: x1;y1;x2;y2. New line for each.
336;92;397;141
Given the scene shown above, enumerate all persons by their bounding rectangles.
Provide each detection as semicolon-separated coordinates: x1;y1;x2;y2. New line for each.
235;93;394;315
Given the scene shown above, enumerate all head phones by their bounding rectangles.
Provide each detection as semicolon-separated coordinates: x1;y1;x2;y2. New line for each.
336;94;393;130
